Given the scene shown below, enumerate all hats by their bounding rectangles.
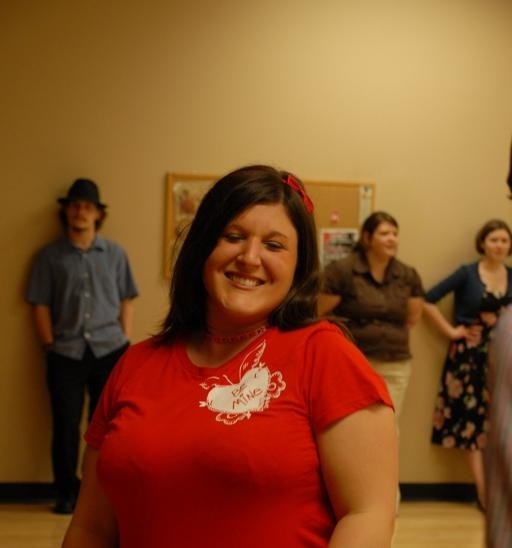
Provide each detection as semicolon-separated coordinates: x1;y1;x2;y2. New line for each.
57;179;106;208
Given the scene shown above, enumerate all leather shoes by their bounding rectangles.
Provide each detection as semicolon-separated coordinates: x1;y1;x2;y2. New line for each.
56;488;75;513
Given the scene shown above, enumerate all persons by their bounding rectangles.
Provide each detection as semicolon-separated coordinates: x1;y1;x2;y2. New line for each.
24;199;138;514
486;304;511;547
423;219;512;514
315;213;424;420
61;166;399;548
